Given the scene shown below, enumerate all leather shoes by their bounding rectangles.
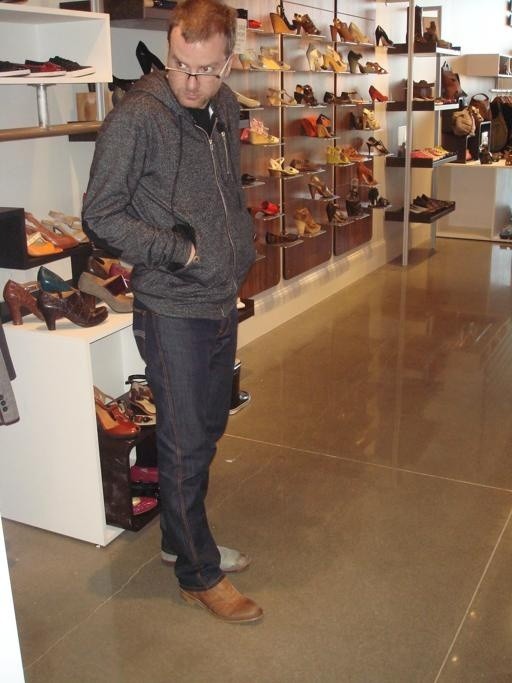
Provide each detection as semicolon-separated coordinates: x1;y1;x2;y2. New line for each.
160;545;251;574
92;373;160;516
403;194;450;216
180;574;263;624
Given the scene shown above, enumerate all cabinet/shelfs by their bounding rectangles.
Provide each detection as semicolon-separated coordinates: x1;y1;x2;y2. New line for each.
435;160;512;242
1;201;161;548
231;26;394;269
0;2;113;84
386;41;460;227
462;54;512;80
59;0;177;141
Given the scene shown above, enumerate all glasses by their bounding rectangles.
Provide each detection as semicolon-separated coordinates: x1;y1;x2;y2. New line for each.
164;55;232;84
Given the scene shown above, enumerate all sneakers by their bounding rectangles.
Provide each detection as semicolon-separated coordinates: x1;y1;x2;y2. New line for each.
1;58;96;79
411;96;457;105
397;146;455;161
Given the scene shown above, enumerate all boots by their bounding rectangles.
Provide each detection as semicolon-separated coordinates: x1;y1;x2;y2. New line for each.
228;361;251;415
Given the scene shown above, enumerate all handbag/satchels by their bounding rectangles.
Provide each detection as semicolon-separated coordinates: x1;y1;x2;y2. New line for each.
404;80;434;99
441;62;508;153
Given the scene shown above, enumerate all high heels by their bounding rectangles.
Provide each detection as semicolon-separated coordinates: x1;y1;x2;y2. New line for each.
233;108;391;246
136;41;164;74
233;5;395;108
3;211;135;331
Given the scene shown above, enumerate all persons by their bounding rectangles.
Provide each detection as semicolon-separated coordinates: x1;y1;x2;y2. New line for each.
82;0;264;623
